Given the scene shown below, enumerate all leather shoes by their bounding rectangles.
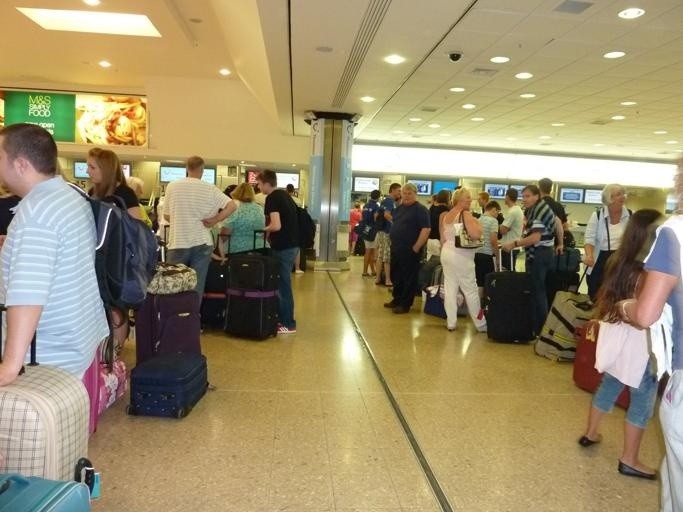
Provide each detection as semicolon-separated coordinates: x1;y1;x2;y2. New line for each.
579;432;605;447
619;457;659;479
384;283;394;287
375;281;385;285
574;321;632;412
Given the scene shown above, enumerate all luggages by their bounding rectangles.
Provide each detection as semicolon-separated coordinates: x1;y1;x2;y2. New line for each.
95;335;128;415
0;471;93;512
485;243;541;342
200;232;232;334
353;237;366;256
534;269;605;363
544;248;579;316
158;225;173;260
227;229;277;340
126;353;210;418
125;292;207;366
0;364;90;482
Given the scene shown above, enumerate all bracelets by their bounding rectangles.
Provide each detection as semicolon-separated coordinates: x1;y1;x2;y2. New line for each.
621;302;631;321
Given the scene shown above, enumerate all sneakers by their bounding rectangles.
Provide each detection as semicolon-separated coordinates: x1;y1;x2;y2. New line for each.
276;325;297;334
294;268;304;274
384;300;399;308
370;272;377;276
362;272;372;276
392;306;409;314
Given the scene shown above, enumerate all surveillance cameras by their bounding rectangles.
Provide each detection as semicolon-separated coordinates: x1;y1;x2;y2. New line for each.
448;50;463;63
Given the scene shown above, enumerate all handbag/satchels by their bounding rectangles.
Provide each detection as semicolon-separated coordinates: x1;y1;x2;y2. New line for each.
149;260;197;295
454;211;484;249
416;255;467;319
353;222;377;242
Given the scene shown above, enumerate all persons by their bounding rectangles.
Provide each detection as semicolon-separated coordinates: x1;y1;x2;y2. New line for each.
161;155;238;333
1;120;113;410
574;207;675;483
582;181;636;299
349;177;568;340
158;168;314;337
612;157;682;512
82;148;145;352
125;175;153;231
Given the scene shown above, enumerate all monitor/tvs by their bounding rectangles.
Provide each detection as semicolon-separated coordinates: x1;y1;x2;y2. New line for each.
584;189;605;204
484;183;510;199
510;184;527;200
354;176;380;193
73;161;91;179
246;171;261;185
200;168;216;185
408;179;432;196
560;187;585;204
120;163;131;178
432;179;459;196
271;172;300;190
160;166;187;183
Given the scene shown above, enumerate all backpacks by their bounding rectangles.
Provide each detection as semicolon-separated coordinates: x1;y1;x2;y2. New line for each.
95;196;156;307
554;229;576;250
282;192;316;248
